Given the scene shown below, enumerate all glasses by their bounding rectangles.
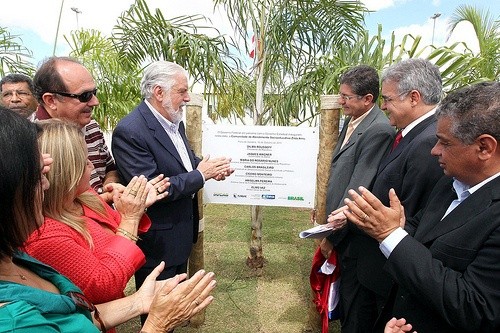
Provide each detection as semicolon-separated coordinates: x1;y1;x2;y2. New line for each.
382;89;411;105
1;90;33;102
41;88;97;105
338;91;360;102
66;291;107;333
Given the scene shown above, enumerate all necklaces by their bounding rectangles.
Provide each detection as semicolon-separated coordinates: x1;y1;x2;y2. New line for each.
0;271;28;281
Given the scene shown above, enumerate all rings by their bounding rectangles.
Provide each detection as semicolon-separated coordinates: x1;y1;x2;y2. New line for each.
193;300;199;305
359;214;367;221
128;189;137;196
156;188;161;194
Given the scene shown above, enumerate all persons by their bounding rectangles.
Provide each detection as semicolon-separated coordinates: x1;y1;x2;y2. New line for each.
0;107;217;333
19;118;171;333
1;74;38;119
384;317;417;333
111;62;237;333
327;59;453;333
32;58;127;210
310;65;396;333
343;80;500;333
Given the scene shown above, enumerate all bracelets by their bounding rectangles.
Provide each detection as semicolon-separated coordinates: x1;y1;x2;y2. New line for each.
116;227;142;242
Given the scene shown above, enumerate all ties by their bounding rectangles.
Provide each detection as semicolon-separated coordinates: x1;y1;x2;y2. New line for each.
388;130;402;155
